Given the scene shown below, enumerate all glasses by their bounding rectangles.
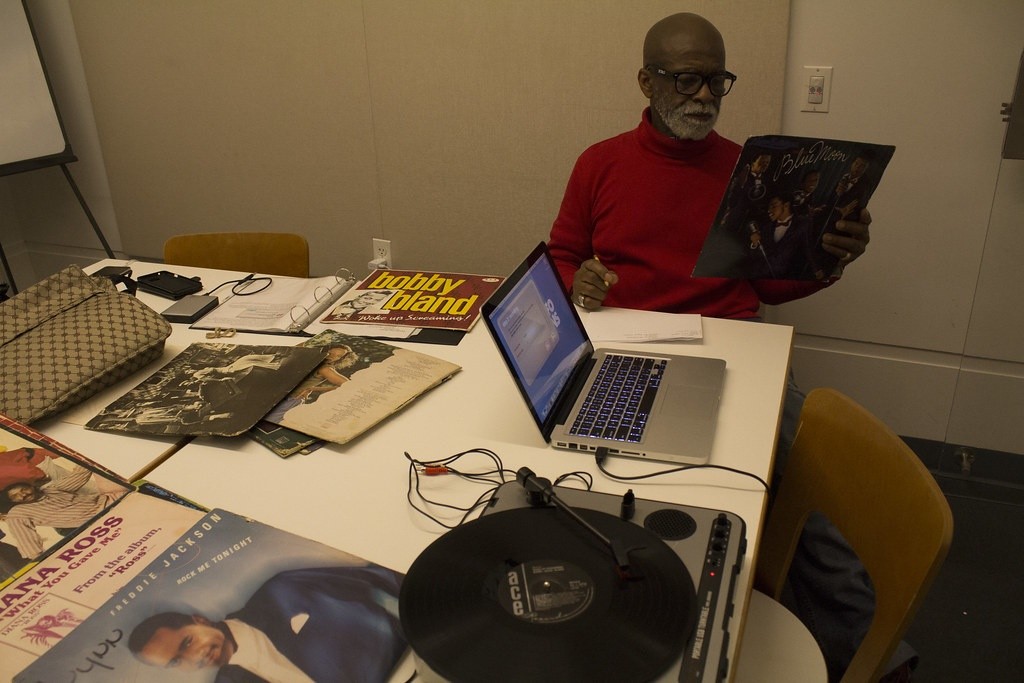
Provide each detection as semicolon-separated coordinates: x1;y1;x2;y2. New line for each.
645;65;737;97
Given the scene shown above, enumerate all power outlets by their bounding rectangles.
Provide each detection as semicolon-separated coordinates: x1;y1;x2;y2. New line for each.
373;237;391;269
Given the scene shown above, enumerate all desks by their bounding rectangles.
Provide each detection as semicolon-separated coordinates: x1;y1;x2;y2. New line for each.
1;254;795;683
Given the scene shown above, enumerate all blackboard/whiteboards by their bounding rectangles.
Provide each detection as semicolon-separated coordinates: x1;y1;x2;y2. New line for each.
0;0;79;176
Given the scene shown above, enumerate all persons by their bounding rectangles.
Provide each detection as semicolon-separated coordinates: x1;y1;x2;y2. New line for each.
5;468;126;562
263;341;351;423
334;290;392;315
184;352;281;383
128;565;412;683
546;11;919;683
738;147;871;279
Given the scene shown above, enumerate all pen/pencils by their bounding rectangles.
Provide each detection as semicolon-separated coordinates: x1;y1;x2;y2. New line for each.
593;254;609;287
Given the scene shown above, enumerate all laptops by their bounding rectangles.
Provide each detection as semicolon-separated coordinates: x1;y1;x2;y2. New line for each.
480;241;727;466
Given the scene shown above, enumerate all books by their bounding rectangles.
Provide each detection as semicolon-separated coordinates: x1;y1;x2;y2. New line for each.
690;135;896;281
0;415;418;683
84;266;504;459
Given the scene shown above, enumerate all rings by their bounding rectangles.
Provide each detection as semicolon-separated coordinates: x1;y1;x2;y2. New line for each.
841;251;851;261
578;296;585;307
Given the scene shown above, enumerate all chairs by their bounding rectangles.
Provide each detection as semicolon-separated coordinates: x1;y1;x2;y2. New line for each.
735;387;954;682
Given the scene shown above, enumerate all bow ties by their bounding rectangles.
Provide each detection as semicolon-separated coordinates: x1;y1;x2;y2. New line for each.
800;195;808;199
754;176;761;180
775;222;789;227
847;180;854;184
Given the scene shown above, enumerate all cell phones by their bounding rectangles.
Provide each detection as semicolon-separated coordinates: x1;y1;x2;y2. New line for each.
90;266;131;280
